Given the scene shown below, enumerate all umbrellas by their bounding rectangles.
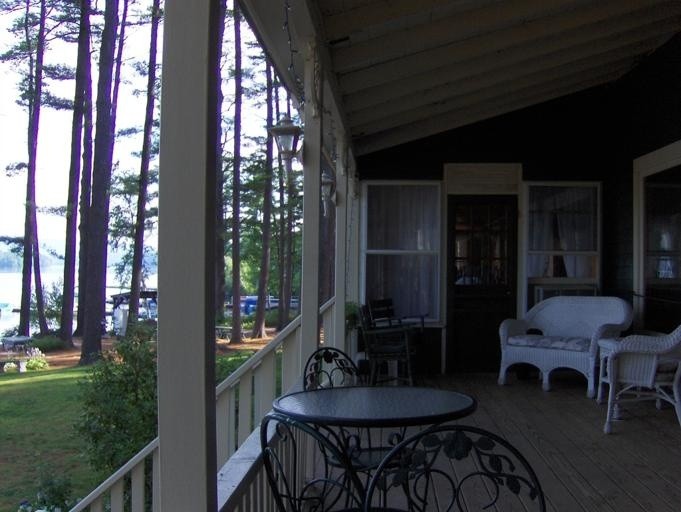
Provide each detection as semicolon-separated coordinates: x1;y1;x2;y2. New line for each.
110;291;157;309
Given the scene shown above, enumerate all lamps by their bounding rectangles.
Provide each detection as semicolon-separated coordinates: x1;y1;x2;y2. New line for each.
321;170;337;201
267;112;305;163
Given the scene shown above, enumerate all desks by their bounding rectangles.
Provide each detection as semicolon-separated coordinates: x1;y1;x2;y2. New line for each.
271;386;478;511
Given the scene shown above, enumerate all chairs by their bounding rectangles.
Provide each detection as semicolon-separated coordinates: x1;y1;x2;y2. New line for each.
499;293;681;435
359;297;429;386
365;424;546;512
261;413;366;511
301;346;431;511
